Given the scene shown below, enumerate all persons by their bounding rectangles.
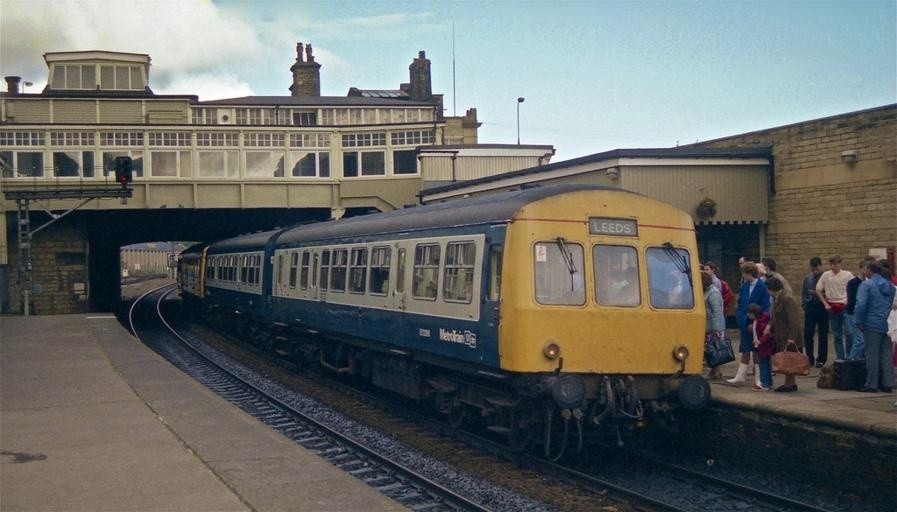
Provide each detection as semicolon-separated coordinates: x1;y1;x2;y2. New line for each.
612;253;897;392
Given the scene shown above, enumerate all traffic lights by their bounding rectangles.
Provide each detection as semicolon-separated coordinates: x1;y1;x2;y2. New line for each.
114;156;134;183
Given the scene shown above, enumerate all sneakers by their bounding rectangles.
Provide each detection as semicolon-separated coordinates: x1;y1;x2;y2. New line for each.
752;385;771;392
775;384;798;392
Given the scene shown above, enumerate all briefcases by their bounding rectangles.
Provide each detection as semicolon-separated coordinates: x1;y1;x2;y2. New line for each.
834;358;867;389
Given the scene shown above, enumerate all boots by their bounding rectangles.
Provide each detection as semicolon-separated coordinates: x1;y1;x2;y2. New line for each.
726;362;748;383
755;363;762;384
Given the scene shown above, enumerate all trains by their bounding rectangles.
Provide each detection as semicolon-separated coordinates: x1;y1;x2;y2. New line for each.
175;179;716;477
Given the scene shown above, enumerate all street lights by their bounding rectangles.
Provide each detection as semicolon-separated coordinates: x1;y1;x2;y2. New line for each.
516;96;526;145
21;80;34;93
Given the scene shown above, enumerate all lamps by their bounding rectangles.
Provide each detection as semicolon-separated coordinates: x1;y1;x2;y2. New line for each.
606;166;620;181
839;148;861;165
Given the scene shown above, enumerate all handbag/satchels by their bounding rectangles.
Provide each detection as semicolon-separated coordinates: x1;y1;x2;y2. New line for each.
705;338;735;367
817;365;833;388
774;342;809;375
